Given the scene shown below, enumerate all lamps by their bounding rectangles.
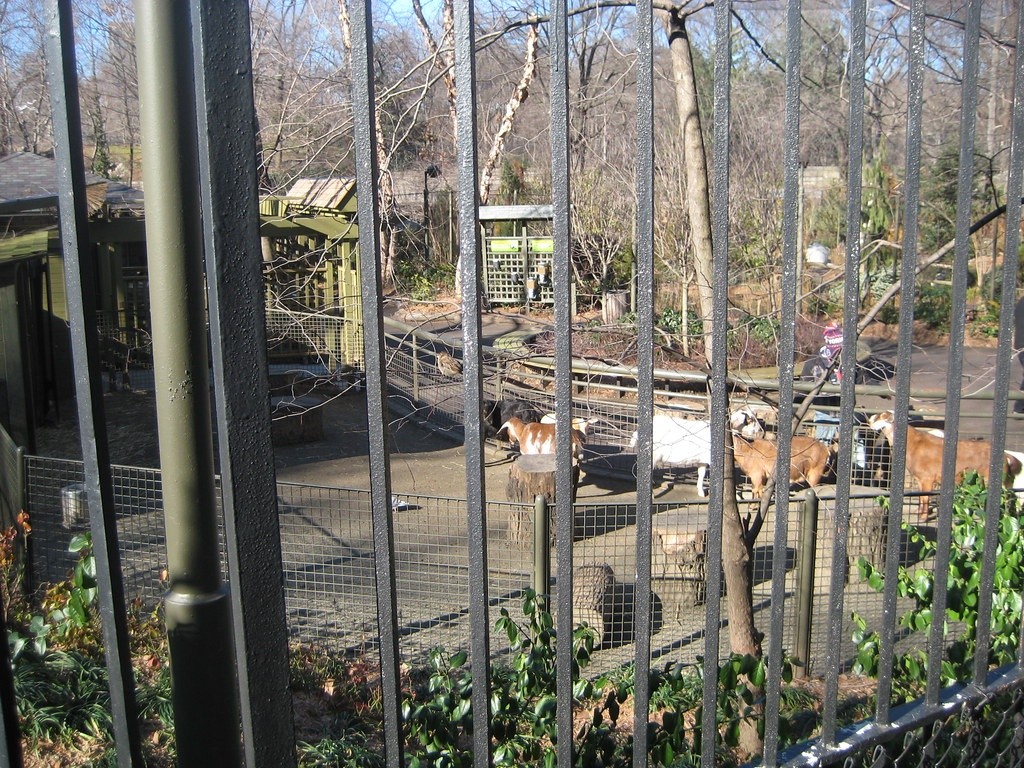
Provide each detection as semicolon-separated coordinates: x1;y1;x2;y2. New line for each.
60;485;91;531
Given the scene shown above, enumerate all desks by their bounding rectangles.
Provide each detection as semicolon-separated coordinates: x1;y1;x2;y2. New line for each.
854;342;871;382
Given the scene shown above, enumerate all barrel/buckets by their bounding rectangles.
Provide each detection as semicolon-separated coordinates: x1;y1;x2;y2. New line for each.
61;484;90;527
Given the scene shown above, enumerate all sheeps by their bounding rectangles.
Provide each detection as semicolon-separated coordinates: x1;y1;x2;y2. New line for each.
482;399;540;452
496;417;585;463
541;414;598;467
868;409;1024;526
732;436;828;508
628;409;765;498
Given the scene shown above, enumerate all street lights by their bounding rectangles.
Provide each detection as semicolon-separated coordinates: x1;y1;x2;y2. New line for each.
424;166;442;283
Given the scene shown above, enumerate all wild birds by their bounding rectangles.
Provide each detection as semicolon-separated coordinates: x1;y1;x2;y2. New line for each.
436;351;463;380
